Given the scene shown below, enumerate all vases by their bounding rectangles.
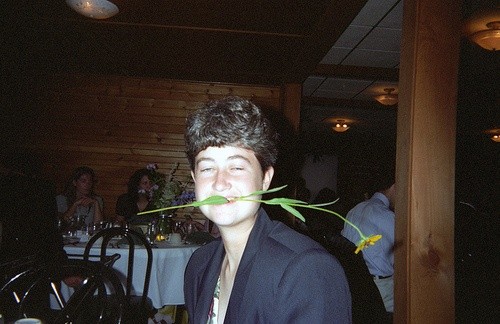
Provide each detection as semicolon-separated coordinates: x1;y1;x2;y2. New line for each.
158;214;171;234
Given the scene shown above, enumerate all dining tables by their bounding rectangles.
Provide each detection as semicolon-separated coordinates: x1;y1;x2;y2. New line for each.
48;224;205;307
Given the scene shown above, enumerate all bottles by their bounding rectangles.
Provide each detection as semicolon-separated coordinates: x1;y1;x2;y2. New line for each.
56;214;122;238
145;223;154;247
159;214;192;237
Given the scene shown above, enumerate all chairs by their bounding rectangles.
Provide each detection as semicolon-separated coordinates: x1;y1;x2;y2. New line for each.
0;226;386;324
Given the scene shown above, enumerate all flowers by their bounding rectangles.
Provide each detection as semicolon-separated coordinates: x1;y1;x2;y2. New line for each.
135;184;384;255
137;160;194;234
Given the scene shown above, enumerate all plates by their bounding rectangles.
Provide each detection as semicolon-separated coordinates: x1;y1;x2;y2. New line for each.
164;241;185;246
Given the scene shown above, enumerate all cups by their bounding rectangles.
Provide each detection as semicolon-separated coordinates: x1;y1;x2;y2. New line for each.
165;233;182;243
14;318;42;324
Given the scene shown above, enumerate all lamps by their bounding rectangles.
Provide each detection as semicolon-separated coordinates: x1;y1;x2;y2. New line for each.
332;119;350;132
468;20;500;51
487;133;500;142
375;88;398;105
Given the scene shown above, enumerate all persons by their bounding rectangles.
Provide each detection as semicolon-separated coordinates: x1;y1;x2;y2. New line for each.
340;173;397;313
0;176;80;308
56;167;103;225
115;168;158;222
300;178;377;233
185;95;351;324
